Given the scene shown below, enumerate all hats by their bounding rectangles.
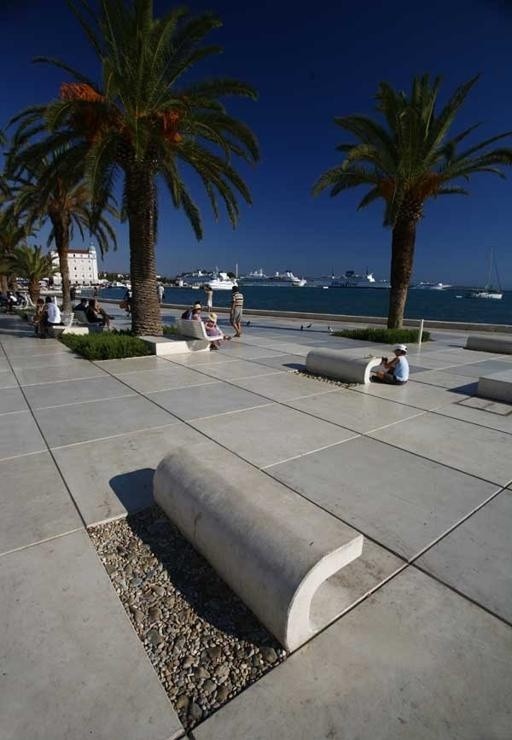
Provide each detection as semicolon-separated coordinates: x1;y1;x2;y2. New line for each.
193;304;201;309
394;346;408;352
208;313;217;321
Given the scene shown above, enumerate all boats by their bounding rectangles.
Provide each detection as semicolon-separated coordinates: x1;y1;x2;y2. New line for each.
20;282;83;296
174;247;391;290
455;295;463;299
108;281;125;288
407;281;453;293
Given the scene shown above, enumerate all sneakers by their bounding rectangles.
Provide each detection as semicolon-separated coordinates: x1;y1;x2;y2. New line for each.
234;330;240;336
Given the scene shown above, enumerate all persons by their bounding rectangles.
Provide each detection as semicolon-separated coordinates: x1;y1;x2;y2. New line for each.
229;286;245;337
157;283;165;304
123;283;133;313
38;296;62;339
86;299;115;331
93;286;98;297
75;298;87;312
0;290;27;315
29;298;45;337
372;344;410;385
181;301;225;350
204;287;214;312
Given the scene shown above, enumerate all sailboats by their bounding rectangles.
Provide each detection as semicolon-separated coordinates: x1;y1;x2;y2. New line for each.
469;248;502;300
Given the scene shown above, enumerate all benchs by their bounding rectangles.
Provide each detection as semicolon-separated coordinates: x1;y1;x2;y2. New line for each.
46;312;74;336
174;318;224;350
74;310;103;332
